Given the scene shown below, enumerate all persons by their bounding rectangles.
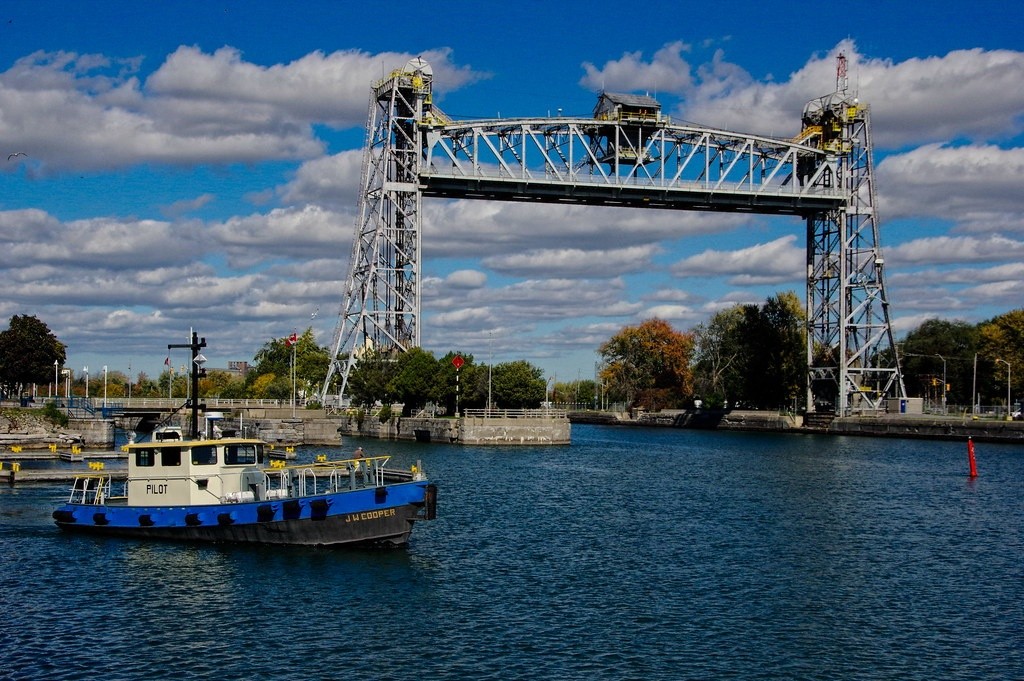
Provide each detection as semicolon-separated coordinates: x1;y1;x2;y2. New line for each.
354;447;365;459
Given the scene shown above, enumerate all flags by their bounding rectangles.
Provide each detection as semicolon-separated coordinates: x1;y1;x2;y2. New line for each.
285;333;296;347
165;358;168;365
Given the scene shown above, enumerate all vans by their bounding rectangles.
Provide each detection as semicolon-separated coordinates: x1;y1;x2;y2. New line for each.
152;427;192;442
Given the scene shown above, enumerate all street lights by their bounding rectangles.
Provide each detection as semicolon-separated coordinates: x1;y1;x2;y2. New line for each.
935;353;947;415
995;359;1011;421
102;365;108;407
83;366;89;399
54;360;58;397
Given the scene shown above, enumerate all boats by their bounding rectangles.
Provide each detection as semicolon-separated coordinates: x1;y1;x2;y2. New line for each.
53;438;438;548
